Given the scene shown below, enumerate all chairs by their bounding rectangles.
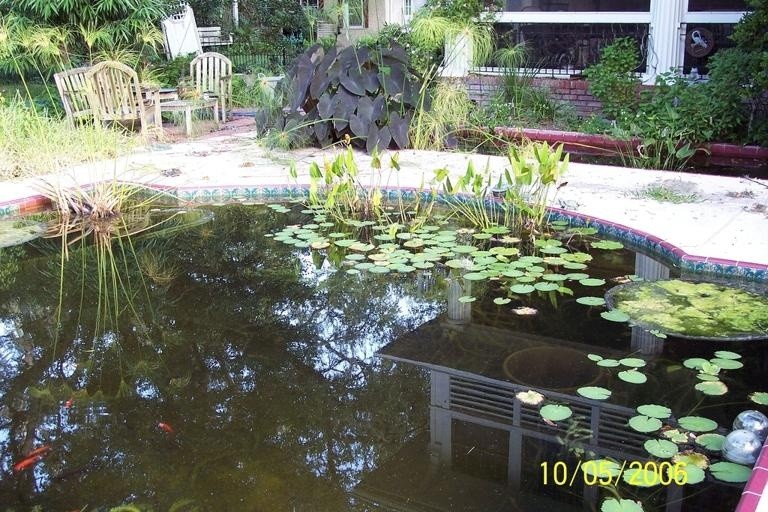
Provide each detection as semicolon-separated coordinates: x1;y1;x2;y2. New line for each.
53;51;232;137
